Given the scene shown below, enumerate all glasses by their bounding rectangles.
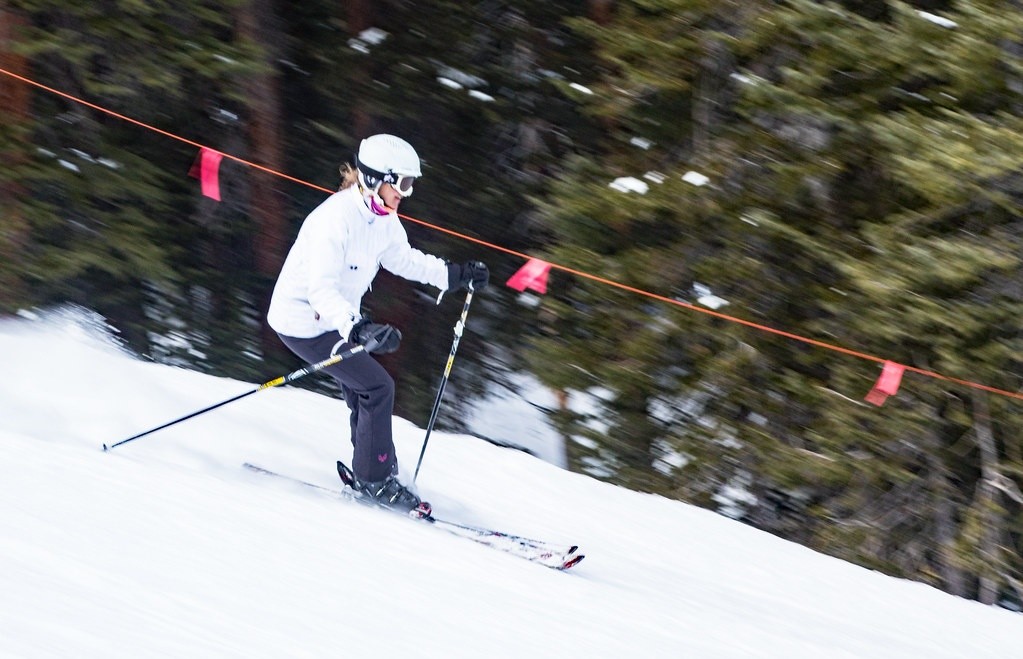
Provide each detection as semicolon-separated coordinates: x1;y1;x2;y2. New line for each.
400;177;413;191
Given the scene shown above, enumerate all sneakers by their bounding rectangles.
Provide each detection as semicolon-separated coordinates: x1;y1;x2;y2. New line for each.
341;472;432;519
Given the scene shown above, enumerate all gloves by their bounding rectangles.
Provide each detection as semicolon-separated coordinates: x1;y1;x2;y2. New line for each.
446;259;489;295
347;318;402;354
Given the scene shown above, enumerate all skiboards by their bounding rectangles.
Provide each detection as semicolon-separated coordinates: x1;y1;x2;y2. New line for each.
242;460;584;574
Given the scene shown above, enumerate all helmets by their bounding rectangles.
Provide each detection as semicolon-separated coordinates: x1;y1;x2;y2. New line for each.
354;131;423;207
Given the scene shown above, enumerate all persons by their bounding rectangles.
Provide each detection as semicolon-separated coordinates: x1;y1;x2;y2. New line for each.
267;134;489;508
